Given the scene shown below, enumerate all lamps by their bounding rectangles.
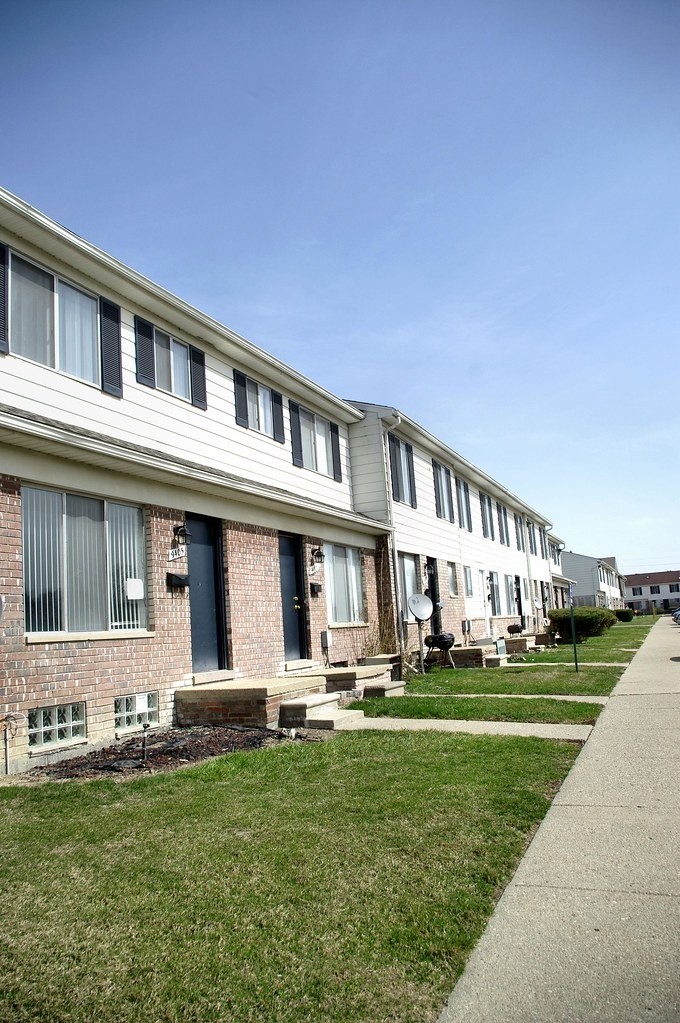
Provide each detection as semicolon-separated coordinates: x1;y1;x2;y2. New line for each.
424;562;434;576
173;522;191;549
311;545;325;563
487;576;493;584
514;581;519;588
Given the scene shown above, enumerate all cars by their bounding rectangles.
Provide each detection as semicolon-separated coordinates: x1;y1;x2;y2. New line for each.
671;607;680;626
